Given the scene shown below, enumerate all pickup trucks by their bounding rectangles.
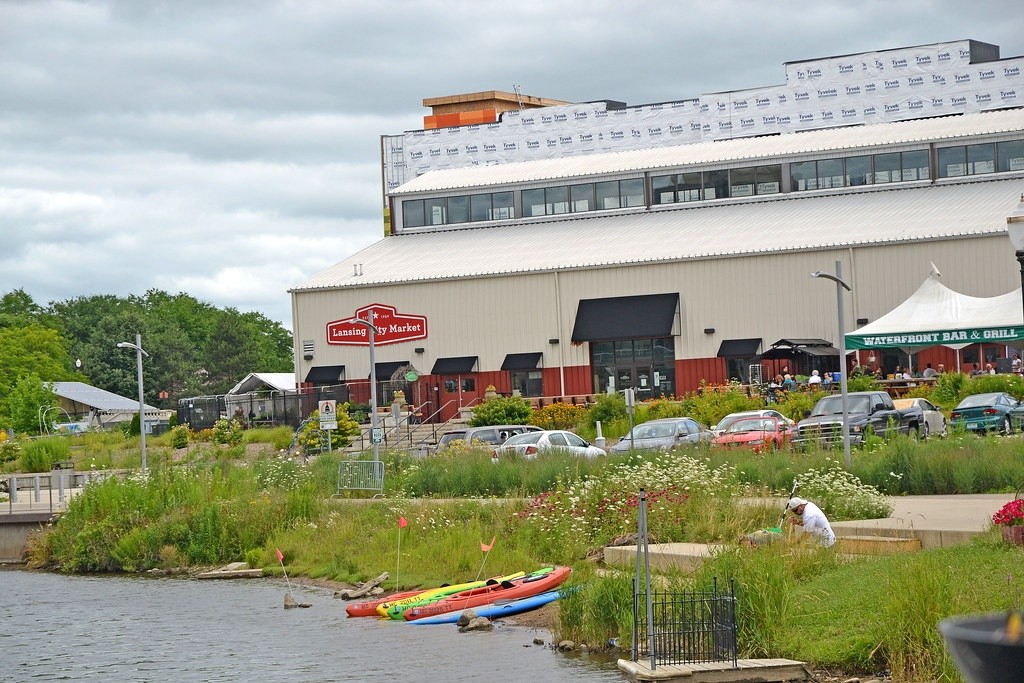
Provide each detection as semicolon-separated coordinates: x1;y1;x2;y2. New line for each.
790;390;926;455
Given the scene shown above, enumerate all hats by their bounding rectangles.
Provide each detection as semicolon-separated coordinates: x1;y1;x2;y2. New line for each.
789;497;807;509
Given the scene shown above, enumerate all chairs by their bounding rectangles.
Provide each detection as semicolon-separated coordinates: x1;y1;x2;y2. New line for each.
385;407;392;412
827;383;840;391
409;405;422;425
916;373;922;378
697;385;760;400
655;427;670;436
930;373;939;378
829;399;841;410
401;406;408;411
511;433;517;436
531;395;598;411
377;407;384;412
784;381;795;389
809;382;822;390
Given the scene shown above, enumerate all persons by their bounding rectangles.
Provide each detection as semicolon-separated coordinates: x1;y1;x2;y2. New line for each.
766;367;834;403
790;497;837;567
848;353;1022;389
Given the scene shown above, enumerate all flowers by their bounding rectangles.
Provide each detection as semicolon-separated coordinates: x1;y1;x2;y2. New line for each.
393;389;404;395
991;498;1024;525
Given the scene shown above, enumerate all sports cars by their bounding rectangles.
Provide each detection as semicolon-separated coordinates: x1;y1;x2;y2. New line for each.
710;417;795;456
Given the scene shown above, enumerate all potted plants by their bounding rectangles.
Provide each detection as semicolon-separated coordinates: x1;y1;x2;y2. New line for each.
484;384;498;404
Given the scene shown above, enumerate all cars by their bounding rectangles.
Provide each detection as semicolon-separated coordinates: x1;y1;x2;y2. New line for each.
609;417;714;456
434;429;467;458
892;398;948;444
710;409;795;438
491;429;608;466
948;392;1024;438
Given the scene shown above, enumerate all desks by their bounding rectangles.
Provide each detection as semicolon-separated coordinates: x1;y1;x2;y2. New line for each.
767;384;782;403
875;376;941;400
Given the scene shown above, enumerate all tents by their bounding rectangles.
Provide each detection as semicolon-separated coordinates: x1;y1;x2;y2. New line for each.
844;276;1024;349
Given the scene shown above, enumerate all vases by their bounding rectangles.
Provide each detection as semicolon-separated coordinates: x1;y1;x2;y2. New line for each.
394;398;406;404
1002;526;1024;546
941;612;1024;683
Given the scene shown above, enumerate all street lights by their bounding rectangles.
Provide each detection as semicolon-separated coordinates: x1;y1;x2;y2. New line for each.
348;309;380;484
810;260;851;468
117;333;149;473
1005;193;1024;333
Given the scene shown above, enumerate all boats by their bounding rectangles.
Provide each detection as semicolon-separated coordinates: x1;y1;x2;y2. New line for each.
348;567;586;625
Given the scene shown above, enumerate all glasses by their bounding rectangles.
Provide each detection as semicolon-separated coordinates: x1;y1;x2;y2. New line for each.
791;506;800;512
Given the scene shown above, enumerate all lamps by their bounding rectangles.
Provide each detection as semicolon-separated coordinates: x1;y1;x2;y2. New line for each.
415;348;424;354
434;383;439;391
304;355;313;361
857;318;868;324
704;328;714;333
549;338;559;344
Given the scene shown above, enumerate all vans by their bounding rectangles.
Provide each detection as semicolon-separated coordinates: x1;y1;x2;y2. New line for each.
464;425;545;451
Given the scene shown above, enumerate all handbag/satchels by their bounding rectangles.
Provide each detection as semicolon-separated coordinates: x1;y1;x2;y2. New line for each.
867;350;876;364
